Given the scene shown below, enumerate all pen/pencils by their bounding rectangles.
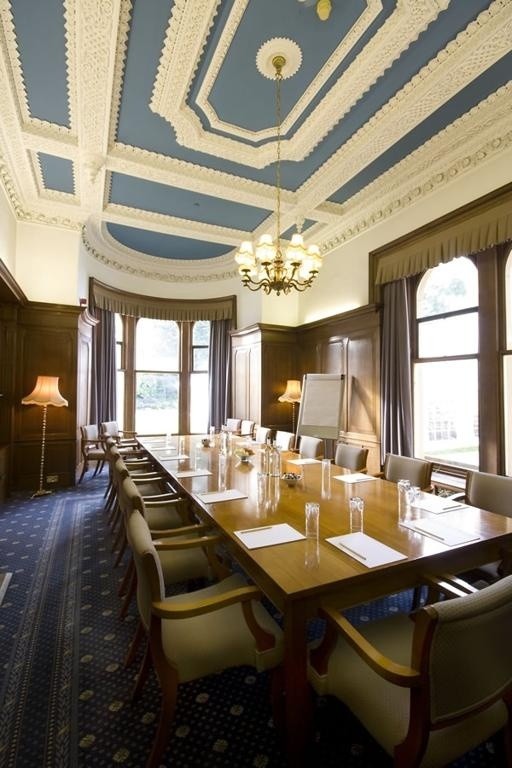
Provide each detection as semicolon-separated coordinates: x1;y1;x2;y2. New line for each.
340;543;366;560
415;526;444;540
443;505;461;510
240;527;272;534
357;478;372;481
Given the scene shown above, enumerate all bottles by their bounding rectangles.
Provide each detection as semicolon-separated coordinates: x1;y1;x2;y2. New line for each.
257;475;281;519
163;424;235;478
264;433;282;476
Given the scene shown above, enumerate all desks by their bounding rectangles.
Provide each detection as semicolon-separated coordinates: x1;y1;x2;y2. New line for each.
133;432;509;767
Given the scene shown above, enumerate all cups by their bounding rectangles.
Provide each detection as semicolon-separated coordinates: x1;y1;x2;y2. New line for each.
303;541;321;569
410;484;422;504
322;457;331;468
301;499;323;540
398;477;410;506
322;469;331;500
348;496;365;533
397;507;422;543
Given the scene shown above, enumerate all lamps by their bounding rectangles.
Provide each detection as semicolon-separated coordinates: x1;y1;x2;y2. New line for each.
276;381;301;433
233;35;324;296
22;374;69;500
